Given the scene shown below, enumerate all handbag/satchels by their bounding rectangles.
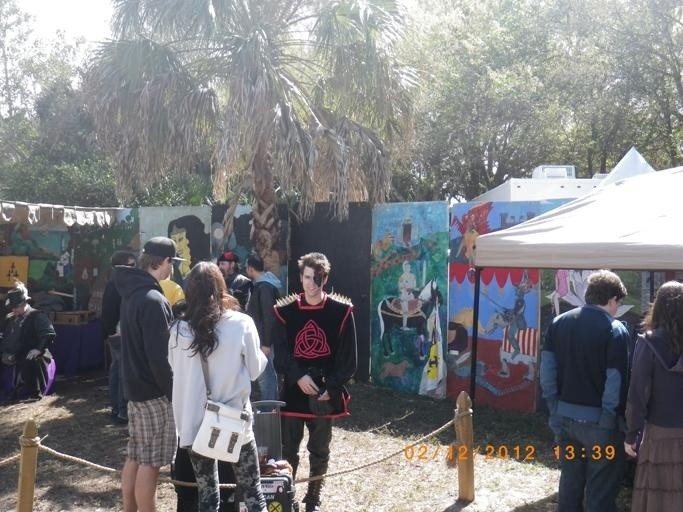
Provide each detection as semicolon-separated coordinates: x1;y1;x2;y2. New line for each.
190;400;251;465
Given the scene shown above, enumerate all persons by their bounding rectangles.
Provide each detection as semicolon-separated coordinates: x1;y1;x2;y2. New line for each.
0;287;57;402
104;238;280;512
624;279;683;512
539;269;632;512
272;252;358;512
551;270;582;317
398;260;418;331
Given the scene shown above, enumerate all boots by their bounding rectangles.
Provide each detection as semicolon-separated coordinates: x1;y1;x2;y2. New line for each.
301;472;326;511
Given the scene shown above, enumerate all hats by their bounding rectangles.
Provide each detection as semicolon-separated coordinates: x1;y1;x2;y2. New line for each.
141;235;185;263
4;287;34;309
219;252;241;263
